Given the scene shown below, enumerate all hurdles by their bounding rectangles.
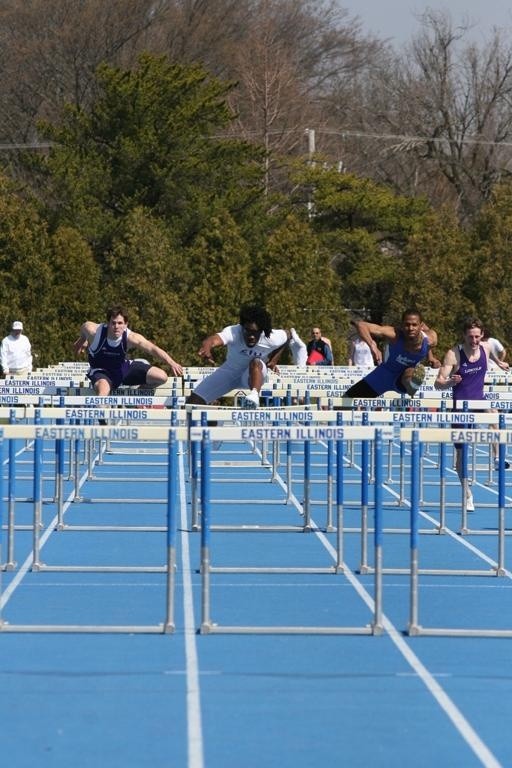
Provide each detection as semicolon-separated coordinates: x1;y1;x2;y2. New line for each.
182;401;319;534
352;409;511;577
26;408;189;573
190;407;352;576
51;396;186;532
318;397;455;535
1;393;52;530
399;426;512;639
0;406;26;571
191;426;394;636
0;422;189;635
457;398;512;535
0;360;512;512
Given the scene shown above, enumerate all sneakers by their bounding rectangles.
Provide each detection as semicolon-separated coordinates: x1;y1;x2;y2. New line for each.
211;421;226;451
465;493;474;512
493;459;509;471
98;418;108;425
407;362;425;395
244;390;260;409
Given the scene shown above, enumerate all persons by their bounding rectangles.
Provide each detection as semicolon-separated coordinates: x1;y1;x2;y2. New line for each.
288;327;309;406
434;318;511;513
480;328;507;362
0;321;34;381
333;308;441;413
348;334;383;411
73;306;185;425
305;326;334;425
186;301;292;451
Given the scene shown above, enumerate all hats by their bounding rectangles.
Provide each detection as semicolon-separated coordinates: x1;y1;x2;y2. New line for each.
12;321;24;330
